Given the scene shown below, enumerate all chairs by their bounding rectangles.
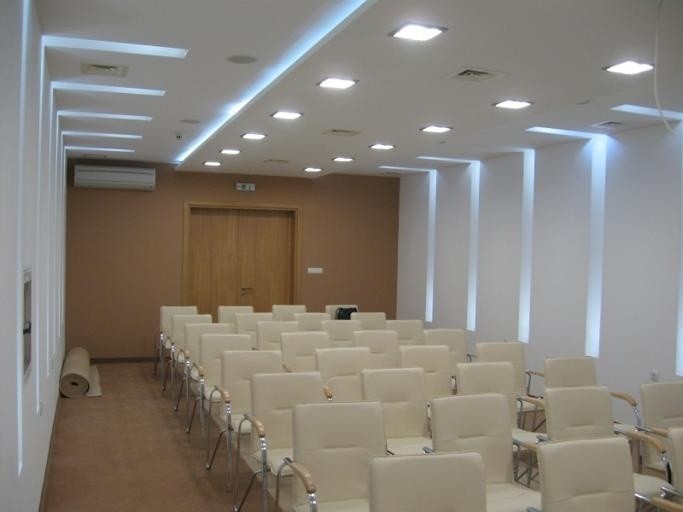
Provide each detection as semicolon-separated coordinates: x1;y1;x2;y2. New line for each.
151;304;639;471
543;384;674;506
526;434;681;511
637;381;682;478
660;425;683;503
370;450;488;511
232;371;334;511
274;400;387;511
430;393;542;512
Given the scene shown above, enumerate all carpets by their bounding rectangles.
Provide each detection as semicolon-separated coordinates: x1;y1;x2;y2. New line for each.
59;347;102;399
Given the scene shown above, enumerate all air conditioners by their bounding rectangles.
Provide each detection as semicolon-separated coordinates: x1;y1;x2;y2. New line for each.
72;164;156;192
